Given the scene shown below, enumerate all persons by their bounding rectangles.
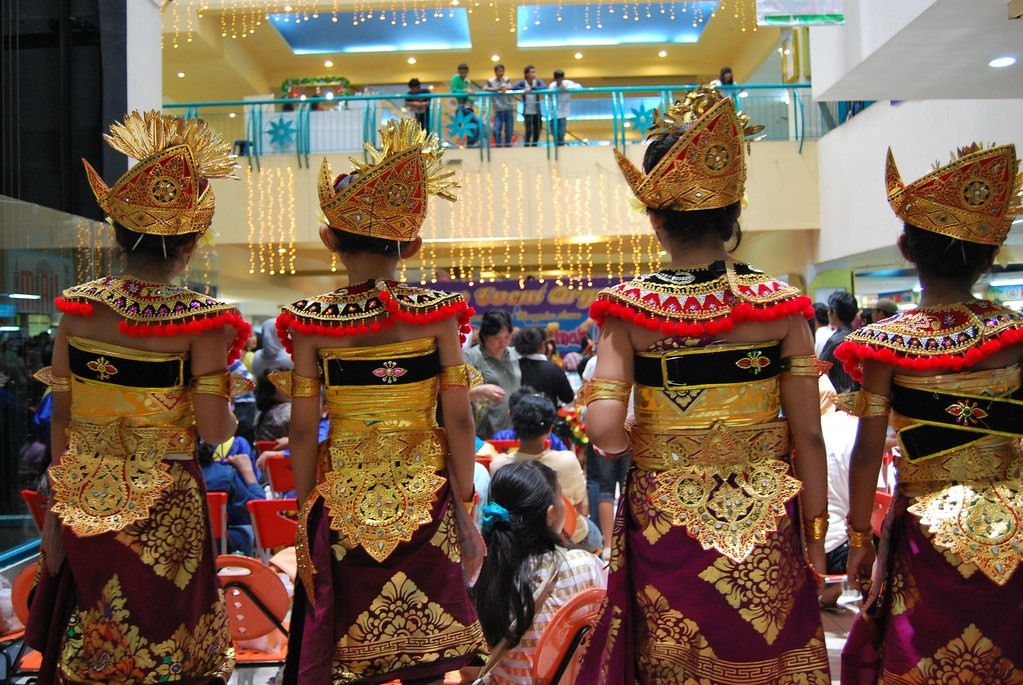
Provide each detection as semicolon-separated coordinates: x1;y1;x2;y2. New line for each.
404;79;430;140
465;460;609;685
450;64;482;148
574;83;833;685
506;65;547;147
833;141;1023;685
274;117;491;685
0;290;899;685
710;67;741;112
548;70;584;147
22;109;256;685
482;65;513;148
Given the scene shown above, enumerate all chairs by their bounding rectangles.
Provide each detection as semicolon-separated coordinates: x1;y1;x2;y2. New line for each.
0;436;608;685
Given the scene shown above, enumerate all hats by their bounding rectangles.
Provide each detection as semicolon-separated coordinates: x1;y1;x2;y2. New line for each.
885;140;1023;246
612;79;766;211
82;108;241;236
867;299;899;315
318;117;463;240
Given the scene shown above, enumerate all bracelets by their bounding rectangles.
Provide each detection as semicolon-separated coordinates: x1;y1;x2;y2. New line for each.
463;492;479;515
845;514;873;548
803;511;829;540
